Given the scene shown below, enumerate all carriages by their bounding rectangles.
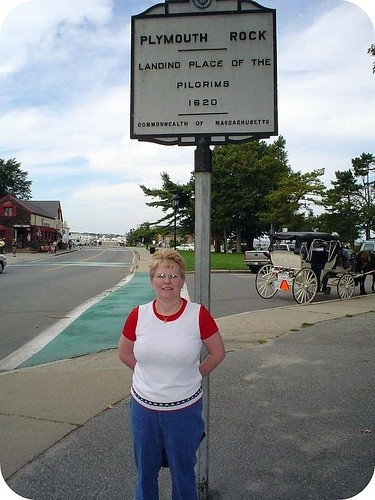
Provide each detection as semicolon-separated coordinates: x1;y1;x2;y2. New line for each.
252;226;375;304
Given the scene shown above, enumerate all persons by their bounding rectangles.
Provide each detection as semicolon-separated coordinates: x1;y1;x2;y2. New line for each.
118;248;226;500
69;240;72;250
12;238;17;258
47;239;58;253
0;238;6;254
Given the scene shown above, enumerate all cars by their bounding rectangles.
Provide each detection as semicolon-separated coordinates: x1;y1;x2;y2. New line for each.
174;242;196;253
72;239;131;248
0;253;8;274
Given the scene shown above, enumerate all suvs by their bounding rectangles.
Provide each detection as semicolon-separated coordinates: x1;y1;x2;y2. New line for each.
358;238;375;252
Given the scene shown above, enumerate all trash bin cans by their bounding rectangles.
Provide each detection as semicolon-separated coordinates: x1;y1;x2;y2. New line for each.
150;246;155;254
146;243;148;250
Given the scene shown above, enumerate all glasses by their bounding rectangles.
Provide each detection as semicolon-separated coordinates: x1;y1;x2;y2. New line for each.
151;273;183;280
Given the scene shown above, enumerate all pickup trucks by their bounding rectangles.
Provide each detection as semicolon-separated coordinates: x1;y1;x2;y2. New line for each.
244;244;303;275
